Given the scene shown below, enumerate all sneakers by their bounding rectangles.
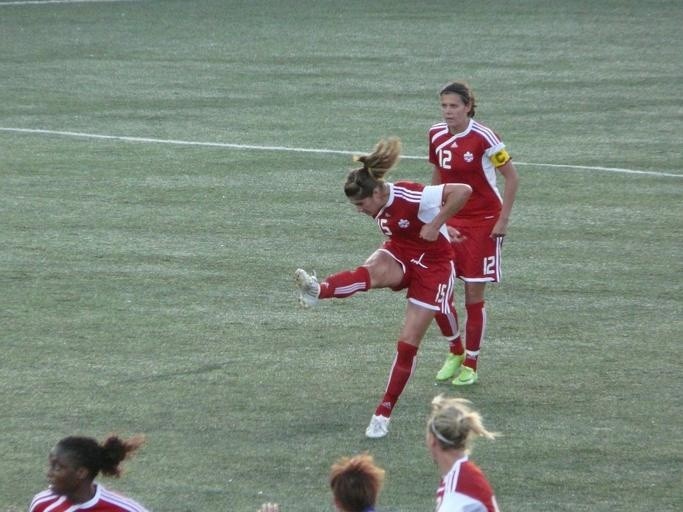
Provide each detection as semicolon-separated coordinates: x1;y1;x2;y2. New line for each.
435;352;478;386
294;268;321;308
365;414;390;438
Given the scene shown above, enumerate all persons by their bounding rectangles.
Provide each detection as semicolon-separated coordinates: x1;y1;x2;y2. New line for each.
257;453;396;512
425;81;519;385
294;134;474;439
425;393;502;511
28;432;152;512
